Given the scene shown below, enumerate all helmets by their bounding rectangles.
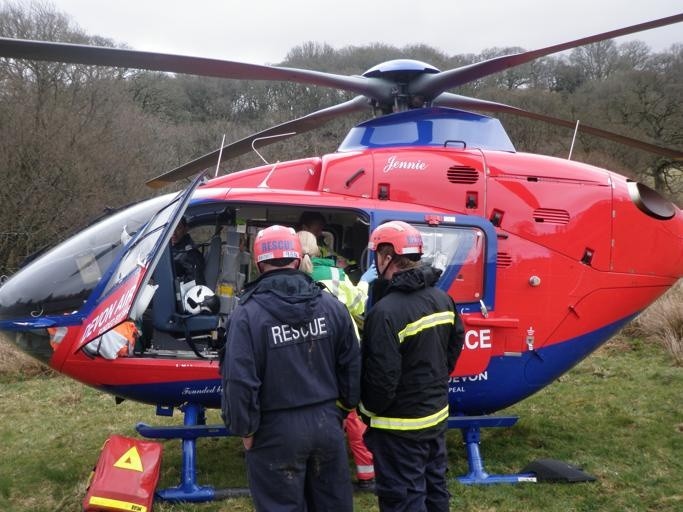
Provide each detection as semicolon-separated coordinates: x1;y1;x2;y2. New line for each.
183;283;221;316
251;224;303;264
366;219;424;257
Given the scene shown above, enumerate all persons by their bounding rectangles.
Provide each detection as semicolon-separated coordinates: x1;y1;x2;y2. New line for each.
170;216;207;286
295;231;378;494
299;211;360;270
218;223;362;511
358;219;465;511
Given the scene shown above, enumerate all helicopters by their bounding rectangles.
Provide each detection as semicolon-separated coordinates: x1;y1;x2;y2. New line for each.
0;14;683;502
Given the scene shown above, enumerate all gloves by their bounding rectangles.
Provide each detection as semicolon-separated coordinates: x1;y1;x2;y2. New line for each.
358;264;380;283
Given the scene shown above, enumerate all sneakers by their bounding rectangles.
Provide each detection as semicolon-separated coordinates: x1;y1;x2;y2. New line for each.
358;477;377;493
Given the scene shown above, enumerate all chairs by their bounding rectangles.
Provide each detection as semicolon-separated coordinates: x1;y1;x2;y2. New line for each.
152;239;218;352
